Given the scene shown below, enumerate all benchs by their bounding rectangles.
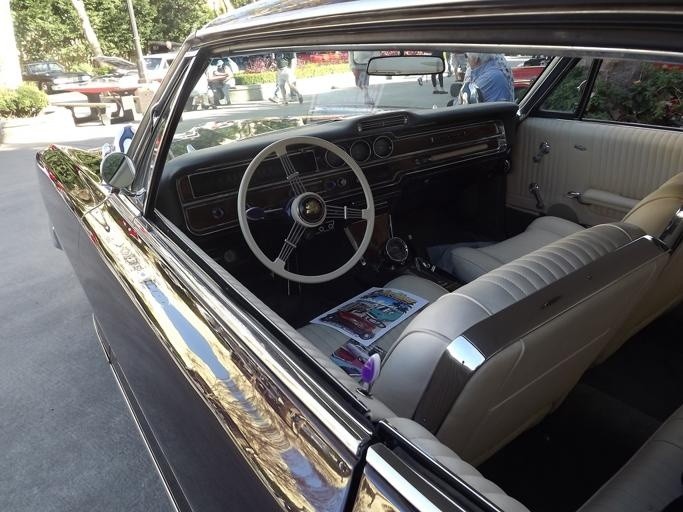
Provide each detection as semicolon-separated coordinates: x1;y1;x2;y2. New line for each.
51;103;115;125
98;96;139;117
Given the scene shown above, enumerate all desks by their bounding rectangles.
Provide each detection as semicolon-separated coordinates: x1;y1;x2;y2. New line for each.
151;76;227;110
64;87;141;125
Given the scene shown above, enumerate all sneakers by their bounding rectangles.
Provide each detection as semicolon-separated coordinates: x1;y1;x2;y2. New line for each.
432;90;448;94
269;94;303;105
418;77;423;86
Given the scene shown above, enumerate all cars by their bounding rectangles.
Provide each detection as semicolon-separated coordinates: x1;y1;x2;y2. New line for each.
28;0;679;512
508;66;547;102
19;41;241;100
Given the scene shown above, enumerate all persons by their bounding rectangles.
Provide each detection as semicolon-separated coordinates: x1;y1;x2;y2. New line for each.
447;51;515;107
429;50;448;95
181;51;304;115
346;49;378;110
447;52;469;81
